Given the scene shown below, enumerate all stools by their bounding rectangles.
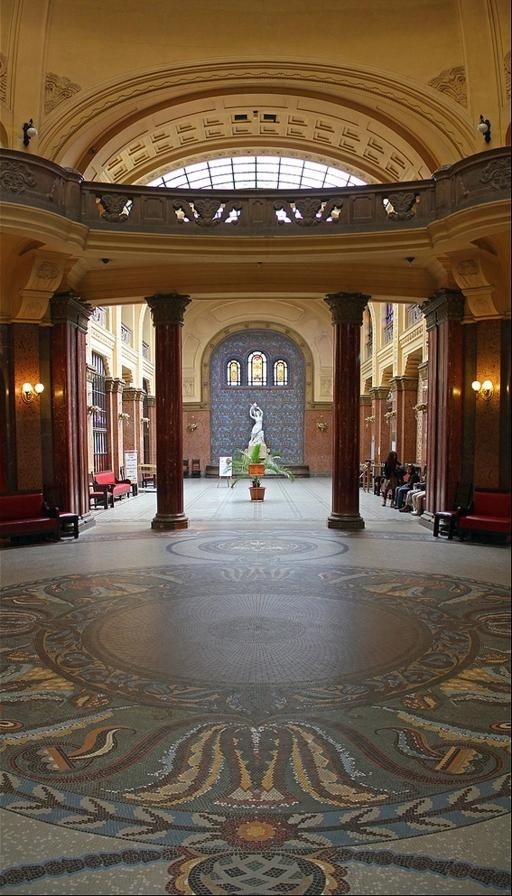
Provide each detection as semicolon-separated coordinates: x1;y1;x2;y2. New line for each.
433;511;458;540
59;511;79;539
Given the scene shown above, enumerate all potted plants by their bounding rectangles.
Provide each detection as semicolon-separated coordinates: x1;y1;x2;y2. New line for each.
247;475;267;502
216;442;294;489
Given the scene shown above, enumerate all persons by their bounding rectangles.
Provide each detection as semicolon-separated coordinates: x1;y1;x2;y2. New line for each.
247;401;264;442
398;464;428;513
381;450;401;508
412;489;427;515
391;463;421;508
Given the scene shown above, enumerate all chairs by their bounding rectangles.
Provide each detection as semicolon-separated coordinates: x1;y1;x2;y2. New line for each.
88;465;138;510
183;458;201;477
1;492;60;543
458;489;511;544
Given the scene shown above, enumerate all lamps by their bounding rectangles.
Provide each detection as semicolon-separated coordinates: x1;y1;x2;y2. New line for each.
21;382;45;404
23;118;38;148
471;379;493;401
476;113;492;143
